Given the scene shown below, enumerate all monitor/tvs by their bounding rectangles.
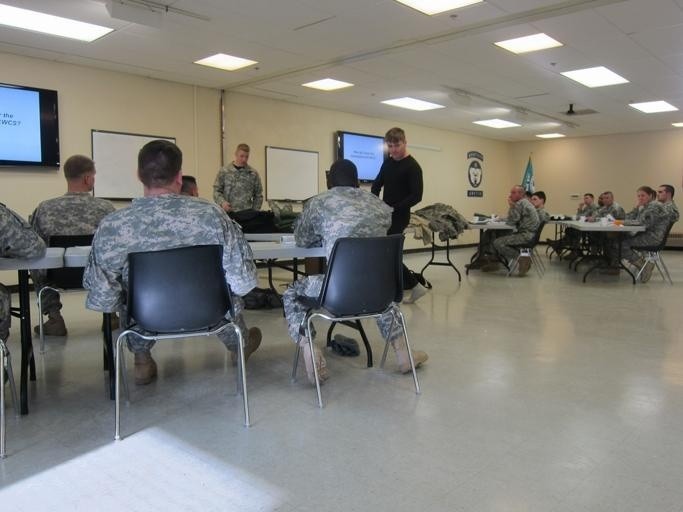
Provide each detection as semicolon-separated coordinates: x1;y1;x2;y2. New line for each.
0;83;60;167
336;130;391;183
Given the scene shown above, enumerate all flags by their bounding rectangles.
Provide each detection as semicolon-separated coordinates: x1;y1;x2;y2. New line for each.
521;152;536;193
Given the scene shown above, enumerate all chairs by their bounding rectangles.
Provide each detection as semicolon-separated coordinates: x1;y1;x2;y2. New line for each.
630;222;674;285
0;338;19;460
115;244;252;441
505;221;547;279
37;234;95;352
290;232;422;407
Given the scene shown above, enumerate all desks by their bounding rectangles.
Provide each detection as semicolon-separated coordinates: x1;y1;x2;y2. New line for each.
64;232;323;268
548;215;645;286
1;245;64;272
420;220;516;280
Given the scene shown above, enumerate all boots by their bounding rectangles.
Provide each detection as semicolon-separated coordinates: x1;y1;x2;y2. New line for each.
631;257;654;283
465;254;532;276
33;311;68;336
133;350;157;386
390;335;428;374
227;327;262;366
101;313;120;331
402;282;428;304
300;336;330;386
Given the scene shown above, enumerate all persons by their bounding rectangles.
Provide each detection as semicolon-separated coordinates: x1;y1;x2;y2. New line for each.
0;203;50;381
464;183;550;276
180;174;199;197
616;185;669;284
656;184;680;224
82;137;264;387
588;190;625;276
371;126;429;306
281;159;430;388
27;153;119;337
546;193;598;260
212;143;264;215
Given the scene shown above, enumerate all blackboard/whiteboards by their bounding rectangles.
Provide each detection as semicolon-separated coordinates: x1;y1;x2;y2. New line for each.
265;146;319;201
91;129;177;201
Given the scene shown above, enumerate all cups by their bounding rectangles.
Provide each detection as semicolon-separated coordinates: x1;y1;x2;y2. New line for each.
491;214;498;219
473;216;479;221
600;217;608;227
580;215;585;224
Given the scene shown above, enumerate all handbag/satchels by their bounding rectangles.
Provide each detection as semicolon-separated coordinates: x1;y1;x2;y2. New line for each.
243;287;282;311
277;211;299;232
227;208;276;233
404;270;426;290
